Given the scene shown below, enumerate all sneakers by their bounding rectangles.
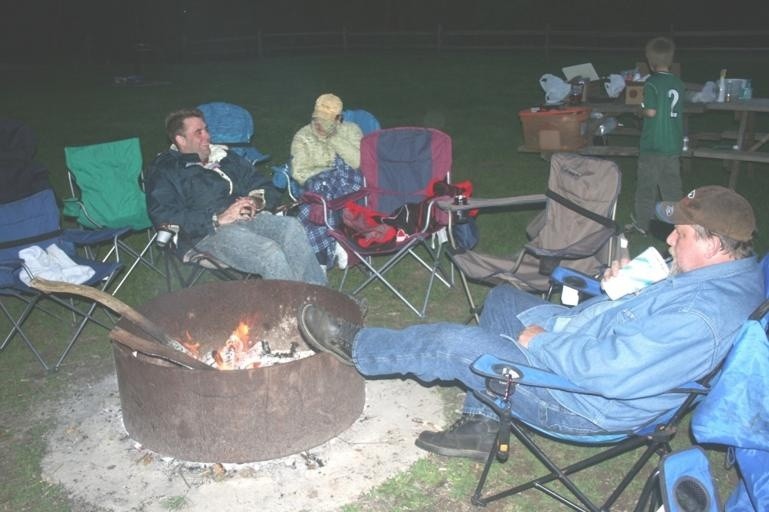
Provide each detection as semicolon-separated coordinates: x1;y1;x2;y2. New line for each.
334;240;349;271
625;223;649;237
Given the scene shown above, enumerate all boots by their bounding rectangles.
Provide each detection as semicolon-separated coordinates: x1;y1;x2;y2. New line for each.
415;412;500;459
299;301;356;364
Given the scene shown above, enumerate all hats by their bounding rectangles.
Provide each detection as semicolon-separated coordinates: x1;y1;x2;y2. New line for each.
656;185;756;241
311;93;342;119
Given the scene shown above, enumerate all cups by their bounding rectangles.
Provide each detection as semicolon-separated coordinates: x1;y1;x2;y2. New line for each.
601;247;671;302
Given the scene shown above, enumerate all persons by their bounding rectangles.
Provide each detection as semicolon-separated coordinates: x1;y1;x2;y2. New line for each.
290;92;365;268
623;35;686;237
144;107;328;287
296;184;765;462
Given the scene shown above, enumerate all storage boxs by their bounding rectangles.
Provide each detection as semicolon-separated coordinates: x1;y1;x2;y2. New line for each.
625;61;682;105
